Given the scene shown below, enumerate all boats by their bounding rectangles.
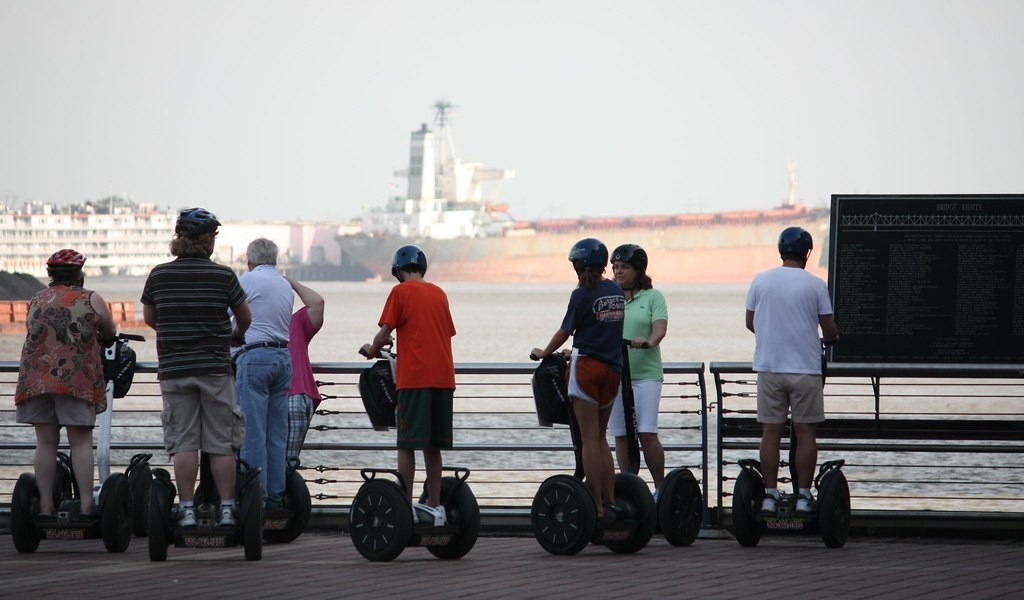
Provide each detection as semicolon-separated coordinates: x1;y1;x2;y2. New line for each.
331;94;836;291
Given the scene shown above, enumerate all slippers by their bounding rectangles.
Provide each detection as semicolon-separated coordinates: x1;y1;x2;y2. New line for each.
38;509;56;522
79;505;98;519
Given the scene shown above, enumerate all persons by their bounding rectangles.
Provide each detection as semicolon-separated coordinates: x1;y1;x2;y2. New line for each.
609;244;668;503
531;238;624;518
362;244;457;524
15;249;116;518
140;208;252;527
227;239;294;510
283;276;325;469
745;227;837;513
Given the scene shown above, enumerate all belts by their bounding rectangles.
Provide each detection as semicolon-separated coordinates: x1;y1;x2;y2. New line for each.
233;342;288;361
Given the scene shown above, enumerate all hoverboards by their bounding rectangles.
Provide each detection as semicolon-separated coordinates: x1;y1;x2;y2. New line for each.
10;332;136;555
527;350;654;555
621;338;702;548
731;336;852;549
349;346;483;558
147;331;265;563
191;462;313;546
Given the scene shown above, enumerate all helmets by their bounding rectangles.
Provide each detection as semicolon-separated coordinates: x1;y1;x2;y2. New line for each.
610;244;648;271
392;245;427;276
46;249;87;272
778;227;813;255
569;238;608;265
175;208;222;236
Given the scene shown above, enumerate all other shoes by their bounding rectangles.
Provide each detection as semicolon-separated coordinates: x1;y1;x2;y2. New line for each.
421;500;447;524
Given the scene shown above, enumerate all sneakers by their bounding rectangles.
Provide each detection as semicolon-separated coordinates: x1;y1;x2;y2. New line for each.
796;493;817;513
217;504;238;525
172;508;198;527
761;493;783;512
